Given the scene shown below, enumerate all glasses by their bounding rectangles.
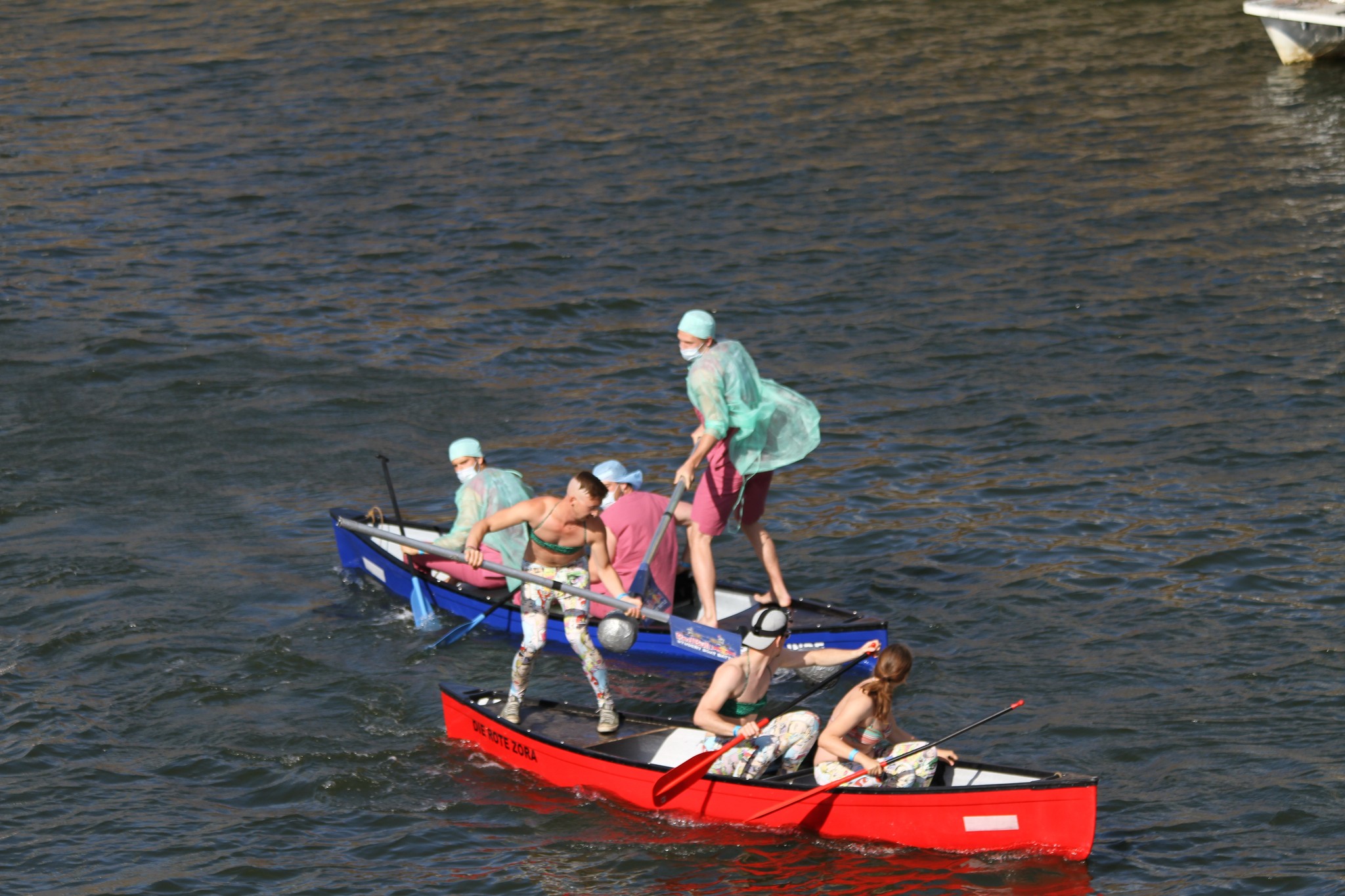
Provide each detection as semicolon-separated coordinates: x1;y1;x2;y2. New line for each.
782;630;792;639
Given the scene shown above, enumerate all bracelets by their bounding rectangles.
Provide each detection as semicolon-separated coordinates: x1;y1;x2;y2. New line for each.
733;725;742;737
418;549;424;554
679;561;691;568
465;546;481;552
848;748;859;761
616;593;629;601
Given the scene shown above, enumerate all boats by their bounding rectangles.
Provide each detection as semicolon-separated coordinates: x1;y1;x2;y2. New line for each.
435;679;1097;863
328;505;895;683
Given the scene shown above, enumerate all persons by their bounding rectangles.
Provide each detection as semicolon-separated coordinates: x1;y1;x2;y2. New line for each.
512;461;694;623
463;470;644;732
673;308;821;630
694;607;881;783
816;644;958;789
400;437;532;599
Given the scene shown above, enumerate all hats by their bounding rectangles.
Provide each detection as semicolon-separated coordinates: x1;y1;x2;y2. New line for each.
678;309;716;340
448;437;485;462
593;460;643;489
741;608;788;650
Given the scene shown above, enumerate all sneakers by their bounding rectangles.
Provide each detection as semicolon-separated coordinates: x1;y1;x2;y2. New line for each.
595;703;620;733
501;696;520;723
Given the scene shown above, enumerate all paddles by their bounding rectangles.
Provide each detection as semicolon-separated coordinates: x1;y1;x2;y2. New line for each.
376;453;443;631
651;644;876;806
426;584;522;649
740;699;1026;824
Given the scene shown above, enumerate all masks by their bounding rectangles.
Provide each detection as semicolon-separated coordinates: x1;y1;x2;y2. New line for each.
455;462;480;483
599;483;623;510
680;343;707;362
